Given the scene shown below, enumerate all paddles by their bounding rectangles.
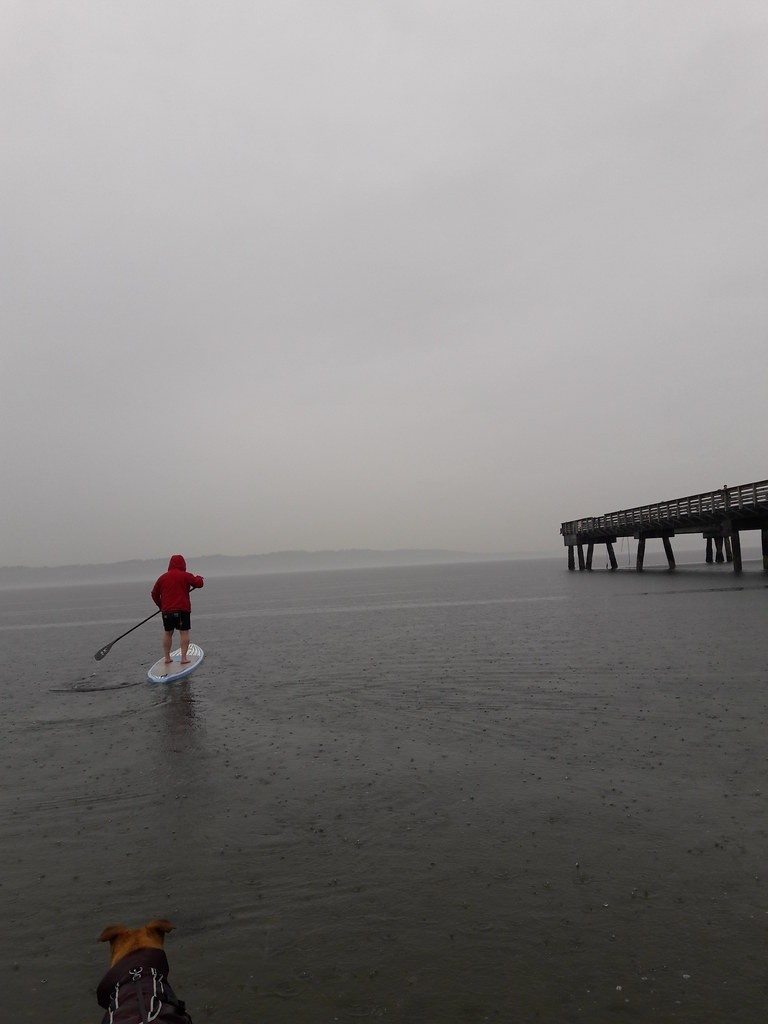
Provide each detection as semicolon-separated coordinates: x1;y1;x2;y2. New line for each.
94;587;197;662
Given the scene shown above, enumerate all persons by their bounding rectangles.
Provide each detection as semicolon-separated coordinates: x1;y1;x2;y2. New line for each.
721;485;731;506
151;554;203;664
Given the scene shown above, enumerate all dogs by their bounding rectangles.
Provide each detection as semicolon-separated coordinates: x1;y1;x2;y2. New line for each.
95;915;193;1024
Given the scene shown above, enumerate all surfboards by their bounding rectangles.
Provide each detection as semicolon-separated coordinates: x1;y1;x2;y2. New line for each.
148;642;204;683
